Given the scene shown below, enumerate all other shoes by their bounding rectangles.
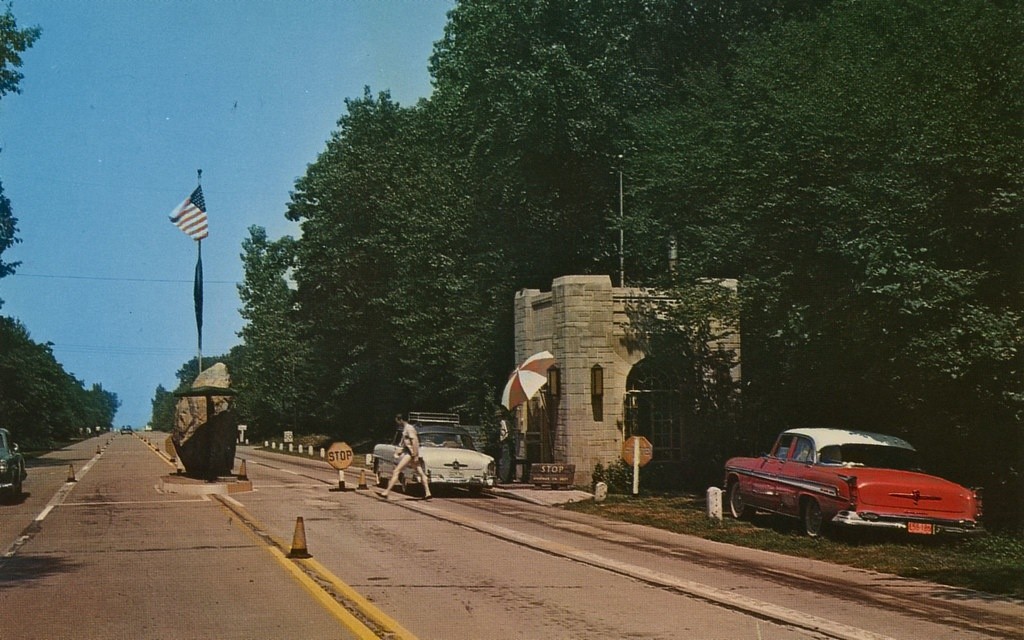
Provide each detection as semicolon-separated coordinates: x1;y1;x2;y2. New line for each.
422;495;432;500
374;492;387;499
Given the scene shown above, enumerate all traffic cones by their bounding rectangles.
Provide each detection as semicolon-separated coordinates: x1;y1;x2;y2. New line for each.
64;462;78;482
238;459;247;480
140;434;151;445
357;470;369;490
155;442;160;451
284;516;314;558
96;444;101;454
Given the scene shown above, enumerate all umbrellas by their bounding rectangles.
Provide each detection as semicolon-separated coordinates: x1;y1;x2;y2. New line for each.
501;349;555;425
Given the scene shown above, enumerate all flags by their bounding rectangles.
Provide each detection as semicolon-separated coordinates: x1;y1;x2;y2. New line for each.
169;182;208;241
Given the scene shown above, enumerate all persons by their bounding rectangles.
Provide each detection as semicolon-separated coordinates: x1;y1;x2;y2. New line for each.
496;410;514;484
374;413;432;502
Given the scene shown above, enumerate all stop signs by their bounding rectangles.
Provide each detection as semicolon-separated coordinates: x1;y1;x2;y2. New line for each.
327;442;354;470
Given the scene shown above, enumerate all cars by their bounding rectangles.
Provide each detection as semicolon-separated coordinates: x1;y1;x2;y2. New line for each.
370;411;498;498
120;425;133;435
725;427;990;543
0;428;27;501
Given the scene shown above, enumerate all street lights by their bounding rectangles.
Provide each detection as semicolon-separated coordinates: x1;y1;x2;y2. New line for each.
617;146;638;288
